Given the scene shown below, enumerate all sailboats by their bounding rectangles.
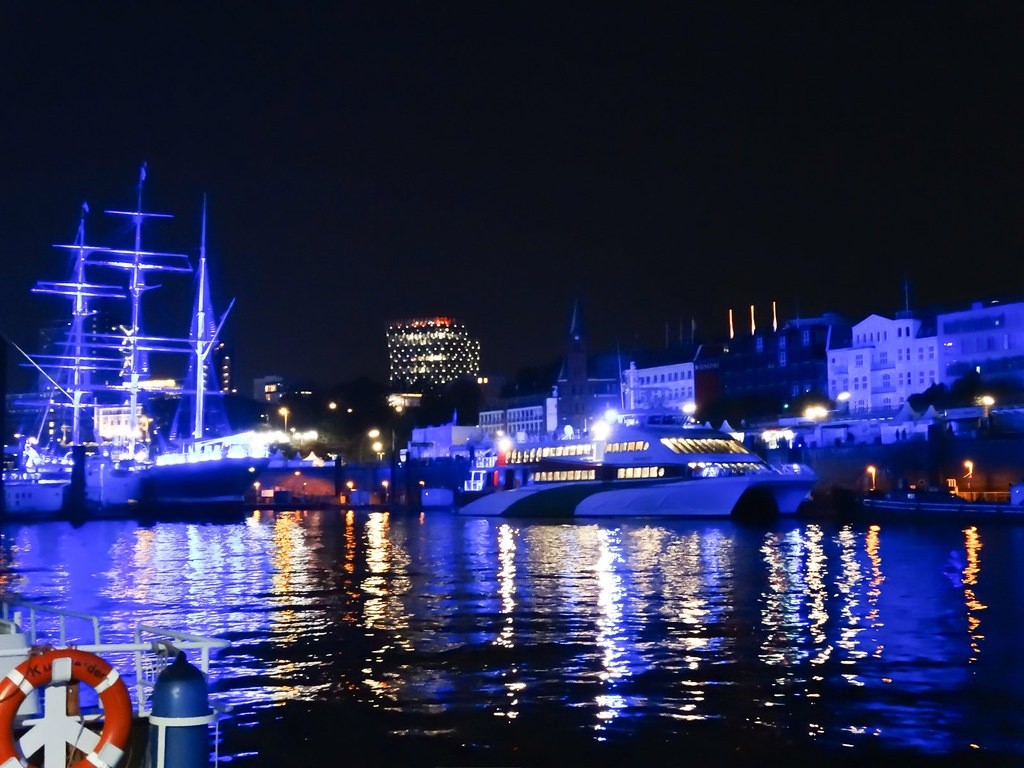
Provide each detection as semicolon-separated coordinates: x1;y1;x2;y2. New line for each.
0;154;306;527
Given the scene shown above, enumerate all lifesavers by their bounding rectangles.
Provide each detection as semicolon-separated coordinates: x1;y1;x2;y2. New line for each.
0;648;134;768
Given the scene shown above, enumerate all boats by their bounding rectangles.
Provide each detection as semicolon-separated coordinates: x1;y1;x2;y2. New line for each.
450;374;823;523
